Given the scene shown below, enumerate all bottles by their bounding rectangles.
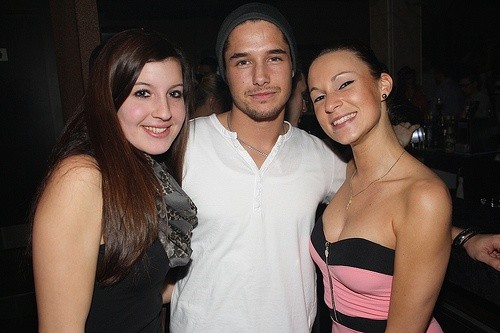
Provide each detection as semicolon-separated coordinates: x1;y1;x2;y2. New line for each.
408;111;458;152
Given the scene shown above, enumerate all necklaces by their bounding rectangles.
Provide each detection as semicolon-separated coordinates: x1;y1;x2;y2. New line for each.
225;109;286;157
345;149;407;210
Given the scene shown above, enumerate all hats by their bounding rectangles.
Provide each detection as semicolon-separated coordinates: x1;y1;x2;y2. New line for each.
216;3;296;84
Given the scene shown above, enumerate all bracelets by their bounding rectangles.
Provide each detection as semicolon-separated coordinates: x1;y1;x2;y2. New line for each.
452;228;472;246
459;231;485;246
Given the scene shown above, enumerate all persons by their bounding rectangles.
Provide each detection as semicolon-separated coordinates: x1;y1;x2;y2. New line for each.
188;58;490;147
27;28;197;333
169;4;500;333
309;43;453;333
